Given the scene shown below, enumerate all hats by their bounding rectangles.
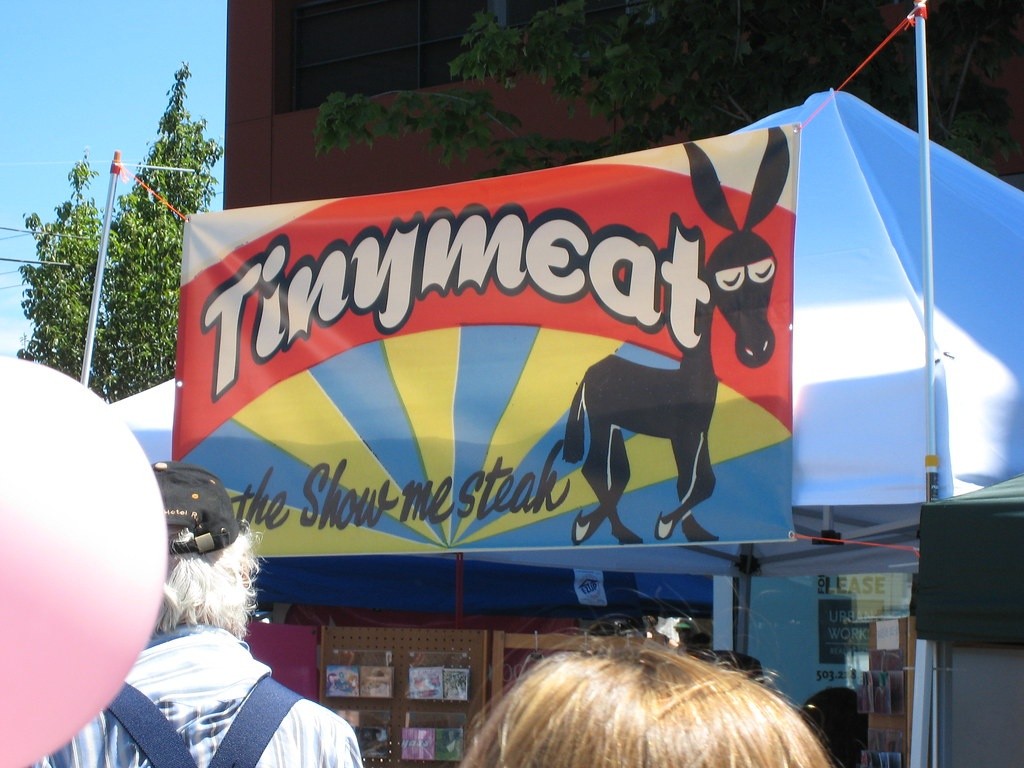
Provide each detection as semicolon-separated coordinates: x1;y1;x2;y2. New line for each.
152;461;240;555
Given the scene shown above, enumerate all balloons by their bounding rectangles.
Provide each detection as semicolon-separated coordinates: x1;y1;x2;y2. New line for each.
0;355;170;768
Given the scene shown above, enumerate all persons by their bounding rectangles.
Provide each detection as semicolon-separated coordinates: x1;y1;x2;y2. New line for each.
457;627;836;768
28;460;367;768
